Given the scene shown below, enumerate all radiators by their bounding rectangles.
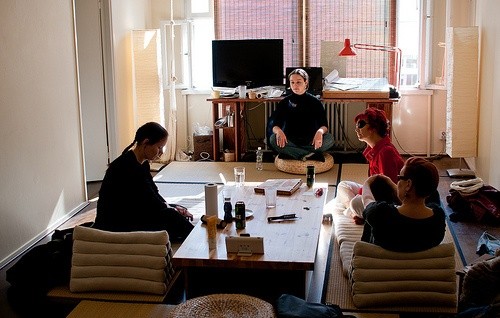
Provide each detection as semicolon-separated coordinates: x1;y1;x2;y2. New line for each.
264;101;348;152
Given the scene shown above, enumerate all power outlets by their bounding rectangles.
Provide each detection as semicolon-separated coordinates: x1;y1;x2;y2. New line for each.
440;130;447;140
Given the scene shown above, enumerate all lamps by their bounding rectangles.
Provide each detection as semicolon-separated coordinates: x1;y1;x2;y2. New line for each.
339;39;402;89
445;26;480;179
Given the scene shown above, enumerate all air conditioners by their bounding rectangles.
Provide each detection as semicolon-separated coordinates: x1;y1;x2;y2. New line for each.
132;29;166;173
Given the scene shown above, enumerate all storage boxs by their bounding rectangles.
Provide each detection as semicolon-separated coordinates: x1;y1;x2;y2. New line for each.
194;132;214;161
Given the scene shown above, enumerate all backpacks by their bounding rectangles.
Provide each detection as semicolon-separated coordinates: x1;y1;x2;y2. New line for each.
445;184;500;228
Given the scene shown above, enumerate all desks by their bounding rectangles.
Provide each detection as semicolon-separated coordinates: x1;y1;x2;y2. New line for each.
206;96;398;161
171;181;328;309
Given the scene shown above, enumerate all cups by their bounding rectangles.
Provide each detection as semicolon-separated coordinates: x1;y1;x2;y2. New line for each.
264;186;277;208
238;85;246;99
249;92;256;100
212;91;220;99
233;166;246;187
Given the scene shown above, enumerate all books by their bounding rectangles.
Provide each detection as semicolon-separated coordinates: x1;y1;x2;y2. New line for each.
254;179;302;195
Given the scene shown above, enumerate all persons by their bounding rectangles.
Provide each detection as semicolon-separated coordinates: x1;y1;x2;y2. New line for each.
91;122;194;244
337;107;405;225
268;69;334;162
361;157;446;253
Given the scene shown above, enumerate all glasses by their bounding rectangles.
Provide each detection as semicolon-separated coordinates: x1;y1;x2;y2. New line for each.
396;175;410;181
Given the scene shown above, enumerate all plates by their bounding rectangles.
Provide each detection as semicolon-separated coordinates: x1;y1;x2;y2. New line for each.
231;209;253;218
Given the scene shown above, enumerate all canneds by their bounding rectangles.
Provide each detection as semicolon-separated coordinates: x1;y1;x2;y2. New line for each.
306;164;315;189
235;201;246;229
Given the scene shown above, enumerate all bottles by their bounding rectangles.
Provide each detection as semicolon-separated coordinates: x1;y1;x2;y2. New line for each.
224;198;233;224
256;147;263;171
235;201;246;229
306;165;315;188
